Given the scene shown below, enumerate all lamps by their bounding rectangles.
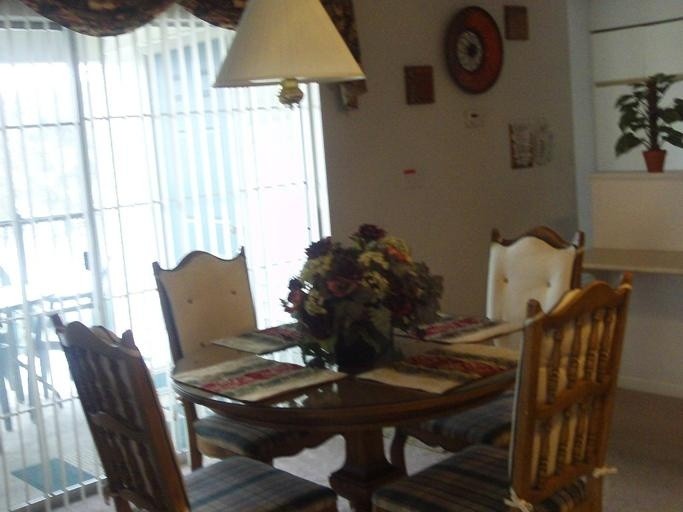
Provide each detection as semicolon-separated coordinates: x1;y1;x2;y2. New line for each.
212;0;365;107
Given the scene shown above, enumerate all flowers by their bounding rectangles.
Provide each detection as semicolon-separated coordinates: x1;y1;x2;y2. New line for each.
277;222;444;371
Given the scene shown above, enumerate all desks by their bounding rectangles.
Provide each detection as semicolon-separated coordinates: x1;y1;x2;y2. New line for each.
175;316;519;512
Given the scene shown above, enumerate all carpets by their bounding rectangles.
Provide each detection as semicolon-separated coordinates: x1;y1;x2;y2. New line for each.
10;457;94;495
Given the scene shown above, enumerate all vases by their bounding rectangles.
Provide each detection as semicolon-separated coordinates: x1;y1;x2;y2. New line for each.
333;305;392;375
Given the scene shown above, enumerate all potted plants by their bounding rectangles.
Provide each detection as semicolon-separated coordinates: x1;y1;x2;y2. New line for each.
614;72;683;172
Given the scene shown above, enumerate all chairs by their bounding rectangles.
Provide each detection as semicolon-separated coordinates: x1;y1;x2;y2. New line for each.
152;246;336;472
50;310;340;512
361;271;632;511
387;223;586;480
